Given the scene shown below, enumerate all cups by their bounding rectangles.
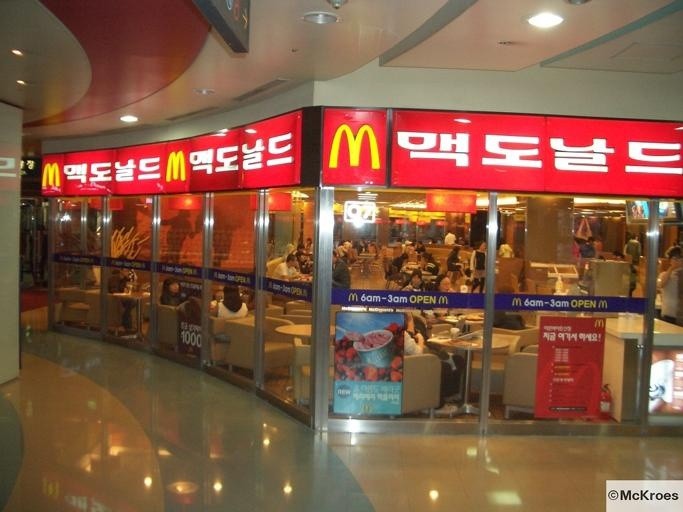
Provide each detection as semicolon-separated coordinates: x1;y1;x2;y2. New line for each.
353;329;395;365
450;328;460;341
585;262;590;270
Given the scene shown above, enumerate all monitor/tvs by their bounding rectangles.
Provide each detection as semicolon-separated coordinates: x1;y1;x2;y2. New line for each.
625;200;683;225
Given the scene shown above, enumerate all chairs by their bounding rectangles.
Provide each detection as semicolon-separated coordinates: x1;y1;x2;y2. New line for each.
291;333;341;405
50;236;682;382
463;329;522;397
505;344;545;420
398;354;438;420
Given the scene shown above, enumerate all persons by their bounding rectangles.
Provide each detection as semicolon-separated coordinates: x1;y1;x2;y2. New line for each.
108;230;683;416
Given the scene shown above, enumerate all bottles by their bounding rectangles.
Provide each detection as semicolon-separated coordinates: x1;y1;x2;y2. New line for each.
556;277;563;293
